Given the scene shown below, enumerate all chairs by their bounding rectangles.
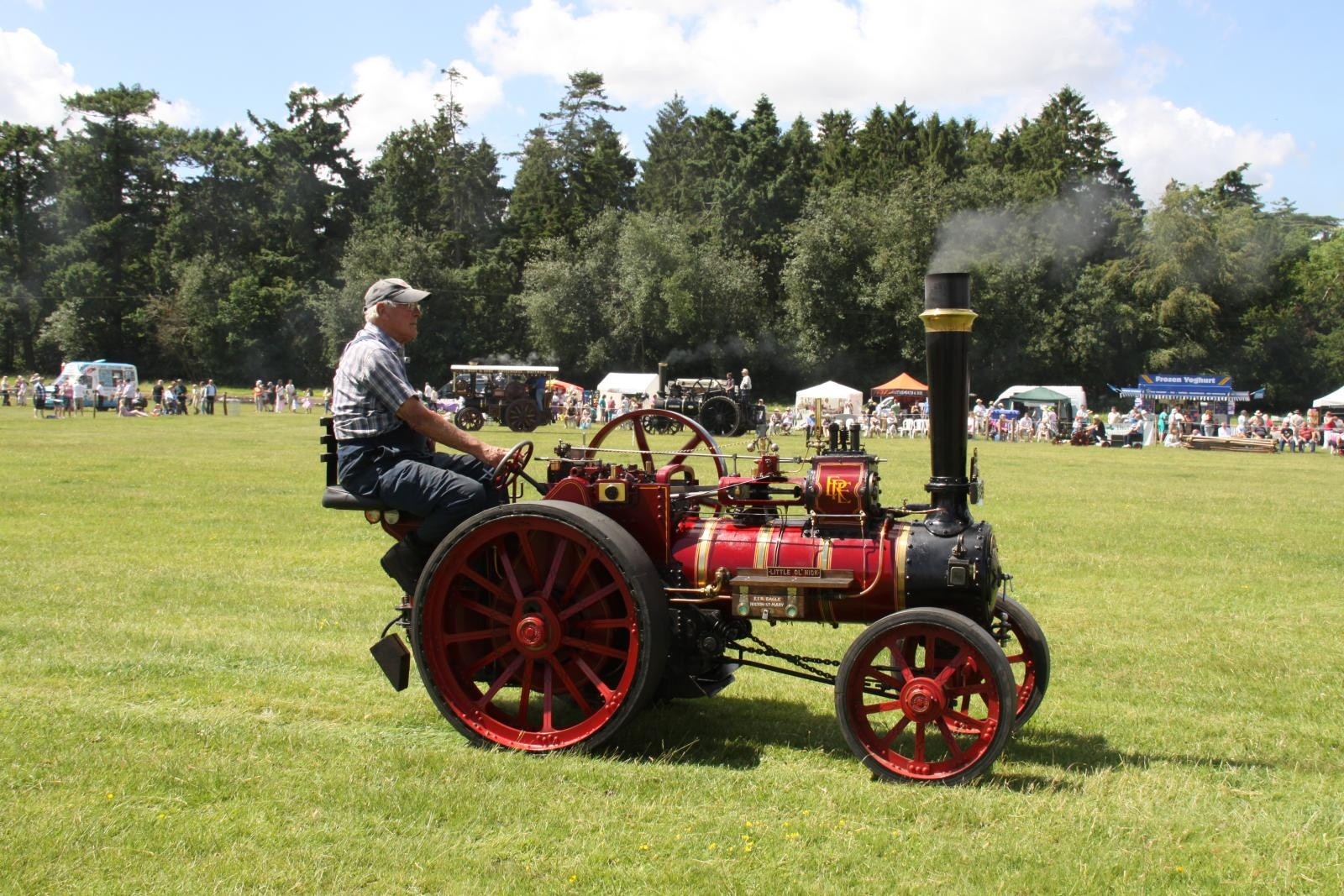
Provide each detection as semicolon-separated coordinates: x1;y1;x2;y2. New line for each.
489;381;525;418
768;413;1052;443
565;406;622;430
702;387;726;402
318;417;399;511
1131;423;1144;448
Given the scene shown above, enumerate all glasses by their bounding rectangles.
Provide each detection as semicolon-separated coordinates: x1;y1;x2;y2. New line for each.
396;302;421;312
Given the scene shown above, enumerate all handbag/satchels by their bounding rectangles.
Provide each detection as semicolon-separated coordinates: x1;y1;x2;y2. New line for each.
17;391;23;395
280;395;285;400
866;409;870;414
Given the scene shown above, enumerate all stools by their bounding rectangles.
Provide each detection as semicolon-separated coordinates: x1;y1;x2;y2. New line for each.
1096;435;1107;442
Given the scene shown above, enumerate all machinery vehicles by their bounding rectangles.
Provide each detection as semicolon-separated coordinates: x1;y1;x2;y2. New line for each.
642;362;768;437
440;365;559;432
1092;429;1144;448
316;273;1050;784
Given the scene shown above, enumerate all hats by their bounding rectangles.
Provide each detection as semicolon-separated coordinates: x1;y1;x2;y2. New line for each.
786;407;790;410
741;368;749;374
1292;410;1301;414
988;401;993;404
976;398;983;403
177;379;181;381
1254;410;1262;416
759;399;763;401
364;278;432;309
997;403;1004;408
868;399;873;402
774;409;779;411
1171;408;1177;413
641;392;647;397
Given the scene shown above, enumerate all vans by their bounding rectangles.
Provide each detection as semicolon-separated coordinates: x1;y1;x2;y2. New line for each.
34;358;138;412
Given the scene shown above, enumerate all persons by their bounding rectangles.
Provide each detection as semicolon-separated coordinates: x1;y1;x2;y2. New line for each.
739;368;752;402
330;277;526;601
0;368;334;420
964;397;1344;456
415;381;441;414
756;395;930;438
527;373;658;431
723;372;735;399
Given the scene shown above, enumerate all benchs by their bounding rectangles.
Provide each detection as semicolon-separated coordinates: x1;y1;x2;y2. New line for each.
1182;434;1277;454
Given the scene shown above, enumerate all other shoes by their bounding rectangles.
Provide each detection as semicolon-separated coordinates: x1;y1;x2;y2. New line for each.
380;538;422;595
1123;444;1131;448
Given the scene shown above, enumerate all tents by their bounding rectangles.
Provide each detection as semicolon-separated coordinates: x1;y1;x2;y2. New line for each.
596;372;660;408
993;385;1088;430
795;380;863;416
544;377;584;401
1313;386;1344;426
1120;373;1250;442
870;372;928;412
